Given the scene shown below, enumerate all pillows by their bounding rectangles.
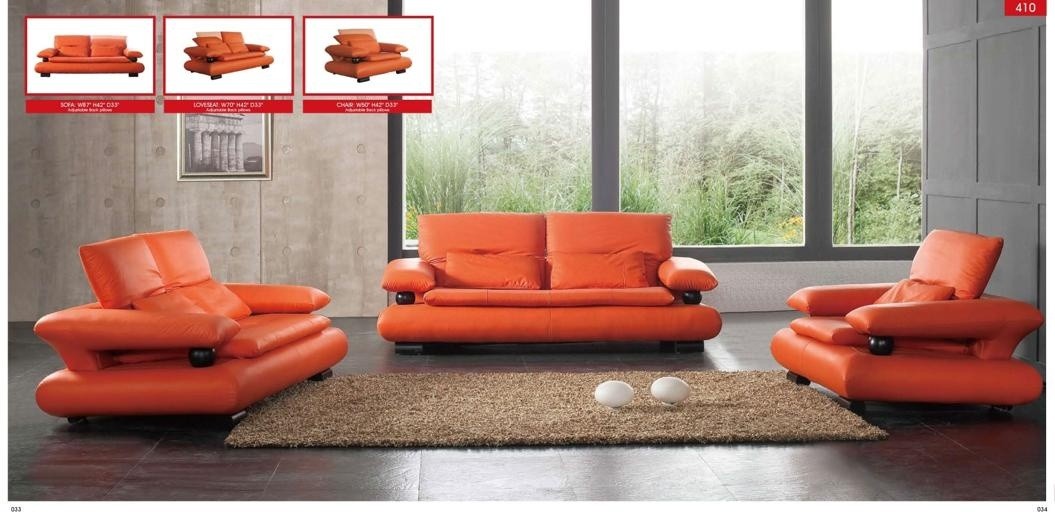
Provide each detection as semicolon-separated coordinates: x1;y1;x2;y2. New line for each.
446;252;546;290
873;279;956;304
206;43;232;54
226;43;248;52
348;40;380;53
546;252;650;289
133;279;251;320
91;46;121;57
59;47;88;56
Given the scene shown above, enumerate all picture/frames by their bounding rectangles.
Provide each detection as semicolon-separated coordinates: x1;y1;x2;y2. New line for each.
177;96;273;182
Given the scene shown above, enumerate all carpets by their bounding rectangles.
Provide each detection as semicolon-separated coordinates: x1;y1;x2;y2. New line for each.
224;371;889;446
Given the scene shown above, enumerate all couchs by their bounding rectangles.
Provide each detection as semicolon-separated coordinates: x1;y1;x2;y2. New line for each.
325;29;412;83
34;35;145;78
377;213;722;354
32;230;348;424
771;228;1044;415
184;32;274;80
